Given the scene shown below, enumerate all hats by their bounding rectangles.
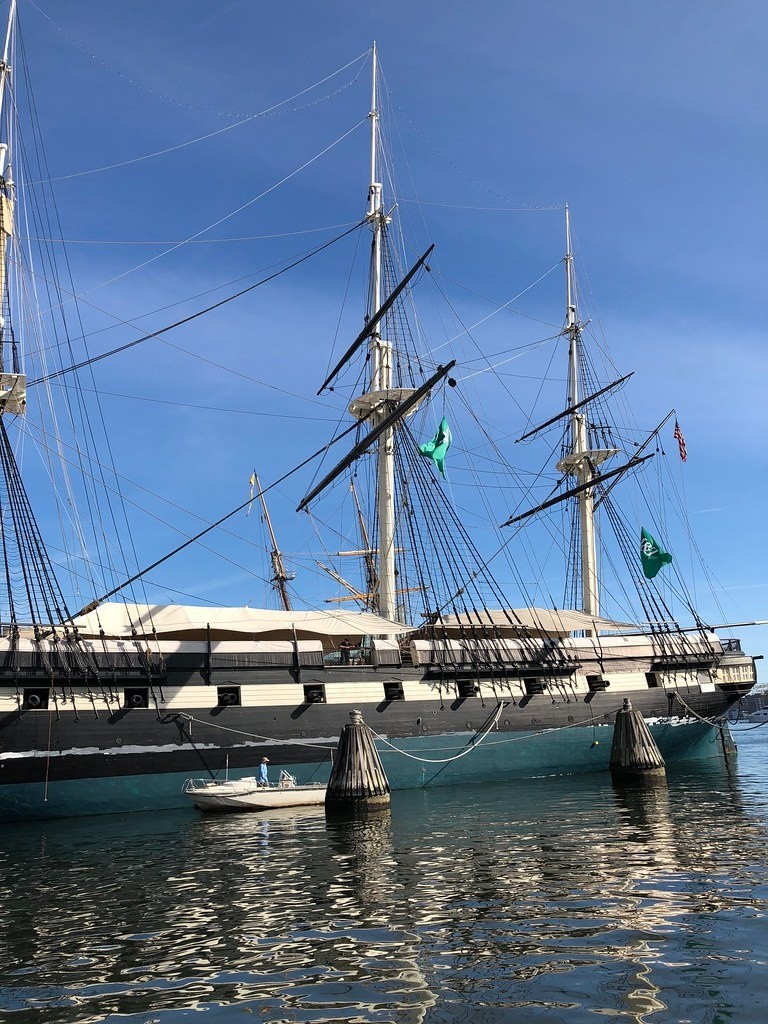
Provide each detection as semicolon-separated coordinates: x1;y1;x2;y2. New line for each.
259;756;270;764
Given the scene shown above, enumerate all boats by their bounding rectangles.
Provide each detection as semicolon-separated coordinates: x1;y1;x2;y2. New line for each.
0;1;768;787
182;776;328;808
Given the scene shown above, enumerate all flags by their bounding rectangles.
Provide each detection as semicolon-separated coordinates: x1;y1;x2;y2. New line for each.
673;417;688;464
639;526;672;579
418;414;453;480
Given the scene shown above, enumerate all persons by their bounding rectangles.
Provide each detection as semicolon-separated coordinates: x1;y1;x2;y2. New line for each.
258;757;270;787
338;638;355;665
